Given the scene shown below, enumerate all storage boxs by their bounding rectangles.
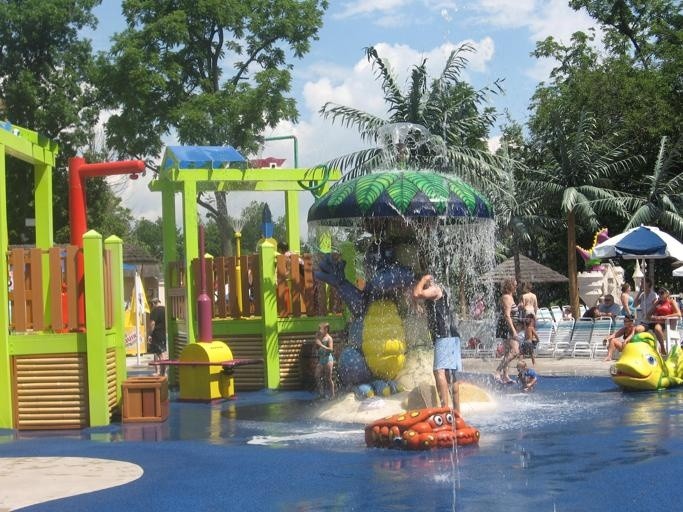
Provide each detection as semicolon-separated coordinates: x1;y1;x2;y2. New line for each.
118;372;169;425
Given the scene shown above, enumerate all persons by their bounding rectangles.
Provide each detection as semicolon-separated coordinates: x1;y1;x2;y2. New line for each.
519;280;538;318
513;359;538;392
519;314;539;367
313;321;335;400
635;288;681;356
487;274;520;384
146;294;165;380
619;283;633;325
602;313;641;361
598;294;620;347
631;275;658;322
412;272;462;413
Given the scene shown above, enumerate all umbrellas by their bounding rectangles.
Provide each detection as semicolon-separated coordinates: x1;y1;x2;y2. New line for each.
590;221;683;323
301;164;497;231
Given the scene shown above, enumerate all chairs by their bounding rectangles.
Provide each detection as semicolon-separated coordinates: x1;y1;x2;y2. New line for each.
457;305;679;359
